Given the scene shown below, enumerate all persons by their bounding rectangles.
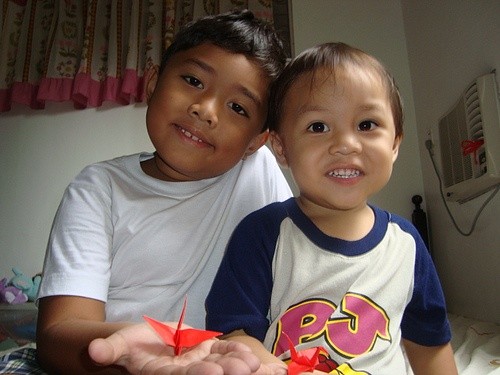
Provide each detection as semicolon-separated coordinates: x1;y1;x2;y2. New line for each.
36;8;294;375
204;41;457;375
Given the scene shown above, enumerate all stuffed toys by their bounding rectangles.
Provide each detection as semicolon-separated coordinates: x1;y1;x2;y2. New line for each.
0;267;41;305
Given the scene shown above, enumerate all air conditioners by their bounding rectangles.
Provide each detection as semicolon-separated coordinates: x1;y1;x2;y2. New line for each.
434;72;500;205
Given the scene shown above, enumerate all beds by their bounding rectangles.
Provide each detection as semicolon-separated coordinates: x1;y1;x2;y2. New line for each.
1;301;500;374
